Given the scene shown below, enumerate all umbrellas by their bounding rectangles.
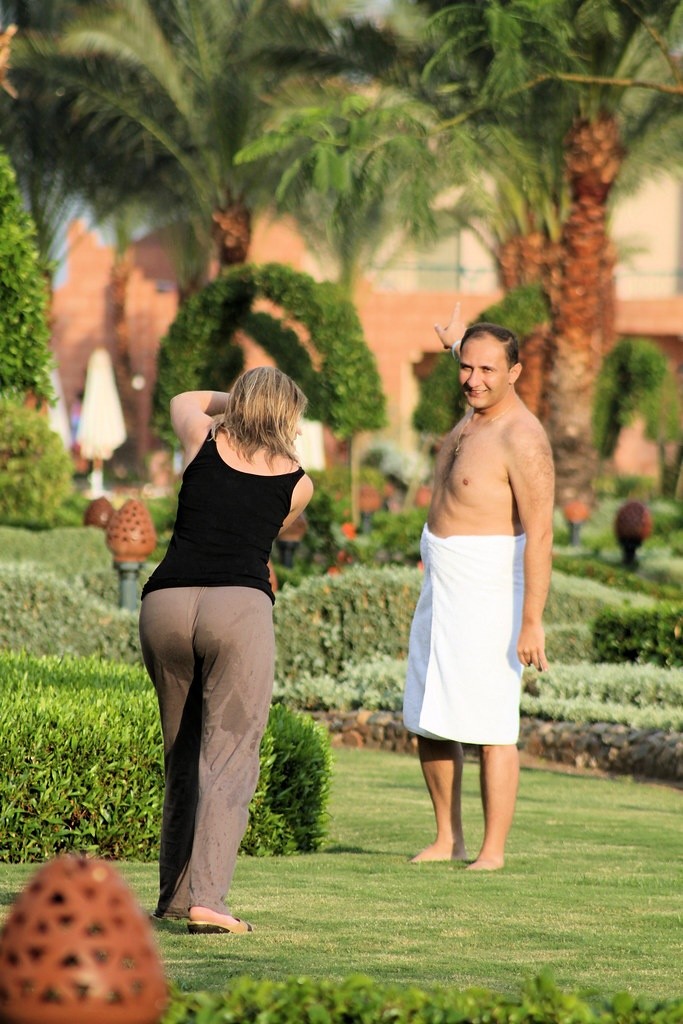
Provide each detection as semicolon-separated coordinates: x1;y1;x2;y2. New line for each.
75;342;129;501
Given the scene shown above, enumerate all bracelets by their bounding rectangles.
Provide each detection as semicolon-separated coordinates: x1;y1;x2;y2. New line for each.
451;340;461;360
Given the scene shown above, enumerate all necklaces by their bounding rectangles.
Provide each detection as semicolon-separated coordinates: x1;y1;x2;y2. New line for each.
455;394;519;453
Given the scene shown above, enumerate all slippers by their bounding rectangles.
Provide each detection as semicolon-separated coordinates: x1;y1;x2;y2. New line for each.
188;915;255;934
150;912;184;925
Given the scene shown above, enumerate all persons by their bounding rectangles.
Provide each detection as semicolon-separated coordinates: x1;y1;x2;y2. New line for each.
400;299;556;872
140;366;312;935
